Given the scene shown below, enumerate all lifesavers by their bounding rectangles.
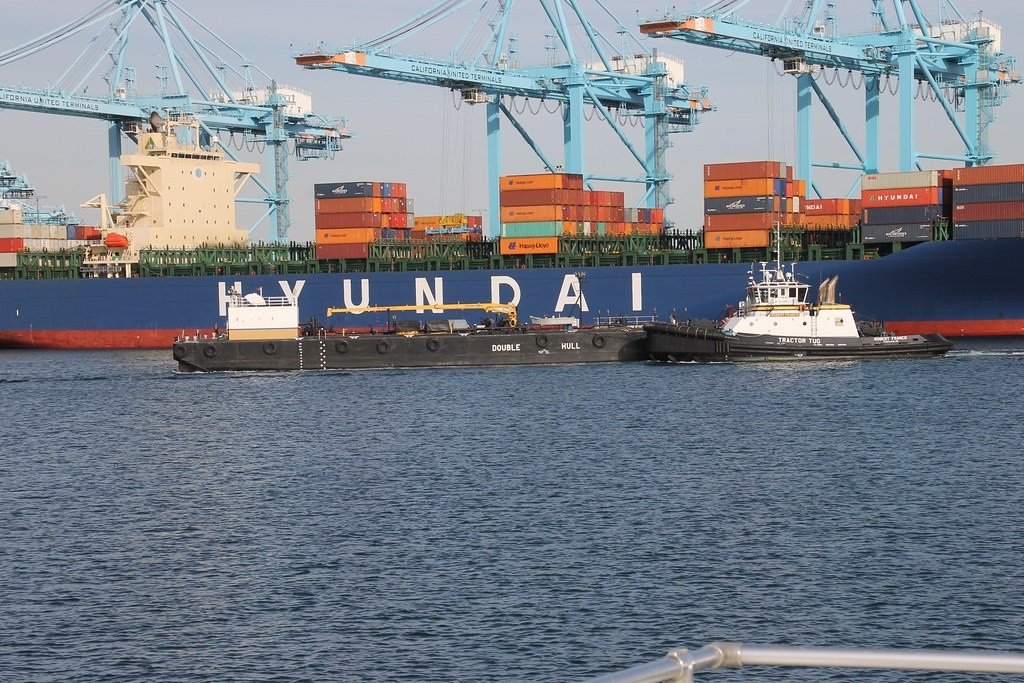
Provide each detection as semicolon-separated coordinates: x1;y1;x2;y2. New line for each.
204;344;218;357
593;334;606;348
263;341;277;354
427;338;441;352
377;340;390;353
536;333;550;348
336;339;350;353
173;344;187;359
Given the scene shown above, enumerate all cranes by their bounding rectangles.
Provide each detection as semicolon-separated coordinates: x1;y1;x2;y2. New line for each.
289;2;718;233
0;2;353;265
637;2;1023;204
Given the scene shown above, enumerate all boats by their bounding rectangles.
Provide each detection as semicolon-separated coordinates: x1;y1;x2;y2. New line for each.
641;220;954;363
